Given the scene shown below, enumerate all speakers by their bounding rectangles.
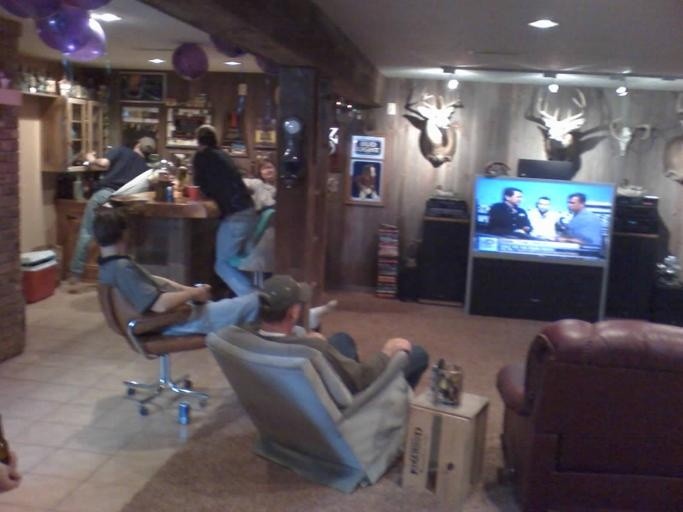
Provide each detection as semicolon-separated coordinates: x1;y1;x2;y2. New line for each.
413;216;477;308
606;230;661;322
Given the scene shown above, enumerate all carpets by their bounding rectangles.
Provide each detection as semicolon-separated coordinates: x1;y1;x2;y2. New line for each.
120;287;553;511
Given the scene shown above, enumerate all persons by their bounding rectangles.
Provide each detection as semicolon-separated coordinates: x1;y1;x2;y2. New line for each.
192;123;258;298
354;164;379;201
90;207;337;336
554;192;602;249
241;153;278;215
526;197;561;240
487;188;534;239
58;134;157;294
237;271;430;395
0;449;22;494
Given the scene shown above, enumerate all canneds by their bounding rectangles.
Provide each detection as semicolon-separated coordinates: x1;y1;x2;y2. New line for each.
165;186;173;202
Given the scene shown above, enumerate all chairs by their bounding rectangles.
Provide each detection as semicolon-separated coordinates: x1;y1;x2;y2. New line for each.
495;317;683;512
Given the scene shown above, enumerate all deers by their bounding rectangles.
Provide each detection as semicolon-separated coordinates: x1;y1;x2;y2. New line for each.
406;94;464;150
535;86;588;154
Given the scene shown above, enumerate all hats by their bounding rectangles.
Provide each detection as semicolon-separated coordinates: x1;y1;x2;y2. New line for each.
253;274;316;315
195;124;217;142
93;206;140;235
136;136;157;156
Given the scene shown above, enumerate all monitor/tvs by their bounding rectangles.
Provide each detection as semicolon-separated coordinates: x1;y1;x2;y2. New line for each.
471;173;617;265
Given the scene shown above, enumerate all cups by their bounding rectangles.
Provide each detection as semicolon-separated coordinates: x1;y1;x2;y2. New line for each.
186;185;201;201
430;363;465;406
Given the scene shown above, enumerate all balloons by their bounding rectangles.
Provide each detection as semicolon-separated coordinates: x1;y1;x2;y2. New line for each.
35;12;90;54
61;1;110;11
255;54;284;74
209;33;249;59
1;1;56;22
170;43;209;83
62;19;108;66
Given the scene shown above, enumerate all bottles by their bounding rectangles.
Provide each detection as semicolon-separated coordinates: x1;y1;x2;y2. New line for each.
20;62;73;96
176;402;189;424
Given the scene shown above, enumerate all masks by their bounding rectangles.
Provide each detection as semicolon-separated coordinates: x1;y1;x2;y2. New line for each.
537;205;550;213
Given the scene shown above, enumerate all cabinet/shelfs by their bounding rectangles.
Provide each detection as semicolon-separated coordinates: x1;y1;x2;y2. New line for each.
41;98;103;173
464;251;609;324
